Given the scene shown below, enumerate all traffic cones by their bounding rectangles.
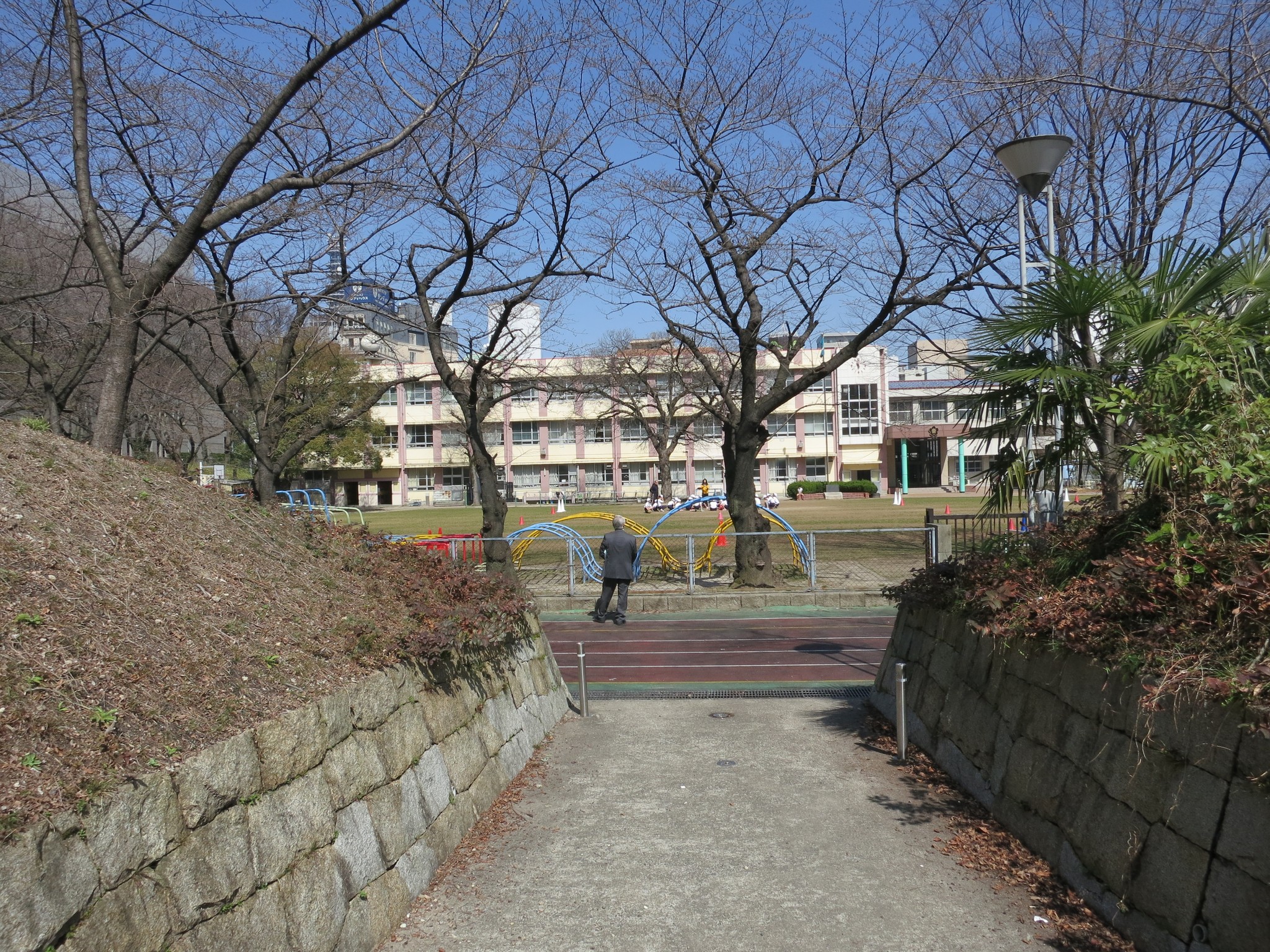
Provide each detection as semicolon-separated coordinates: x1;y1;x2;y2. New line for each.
1020;511;1029;534
713;519;729;547
518;516;525;525
438;527;442;538
944;504;951;514
900;497;905;506
897;492;901;505
561;498;567;512
426;529;434;552
555;499;564;513
892;491;898;505
717;510;724;520
1008;518;1016;530
1074;494;1080;502
1062;488;1071;502
550;506;556;515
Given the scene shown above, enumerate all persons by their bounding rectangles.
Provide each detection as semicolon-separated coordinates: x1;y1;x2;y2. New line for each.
644;498;653;513
593;515;638;625
797;485;805;500
653;493;780;512
700;478;710;508
650;480;659;506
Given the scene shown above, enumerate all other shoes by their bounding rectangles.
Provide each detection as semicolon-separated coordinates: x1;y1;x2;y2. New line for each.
722;509;727;511
612;613;627;625
660;509;664;512
593;614;605;624
766;507;769;510
706;507;709;510
657;508;660;512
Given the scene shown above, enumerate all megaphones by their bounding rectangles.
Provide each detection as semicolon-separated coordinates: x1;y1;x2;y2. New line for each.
993;135;1073;201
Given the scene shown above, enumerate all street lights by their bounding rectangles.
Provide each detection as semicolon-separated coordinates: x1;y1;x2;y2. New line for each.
993;133;1076;527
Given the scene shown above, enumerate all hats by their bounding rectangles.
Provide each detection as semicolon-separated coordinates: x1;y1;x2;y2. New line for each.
762;495;767;499
755;494;758;497
695;496;699;498
655;499;657;502
672;499;675;501
773;493;777;497
767;494;770;496
647;498;651;502
691;494;696;498
675;497;678;500
660;494;663;498
712;499;715;502
688;497;693;500
677;499;681;502
699;495;702;498
